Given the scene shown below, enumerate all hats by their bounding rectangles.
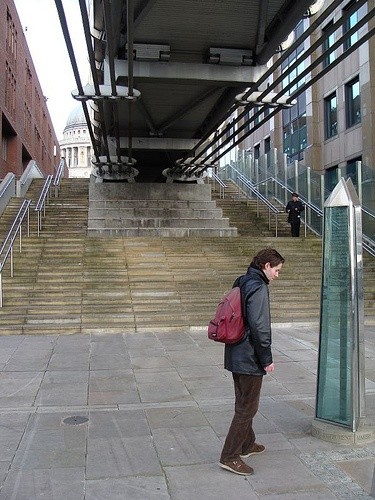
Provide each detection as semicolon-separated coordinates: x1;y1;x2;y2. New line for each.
291;192;299;198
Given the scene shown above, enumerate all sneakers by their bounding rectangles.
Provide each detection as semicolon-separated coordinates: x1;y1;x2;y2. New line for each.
218;442;265;476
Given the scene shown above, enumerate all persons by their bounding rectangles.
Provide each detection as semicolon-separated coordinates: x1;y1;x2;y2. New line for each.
217;248;285;476
286;193;305;237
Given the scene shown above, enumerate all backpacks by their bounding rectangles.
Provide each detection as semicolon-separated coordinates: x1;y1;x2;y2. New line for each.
208;287;245;344
